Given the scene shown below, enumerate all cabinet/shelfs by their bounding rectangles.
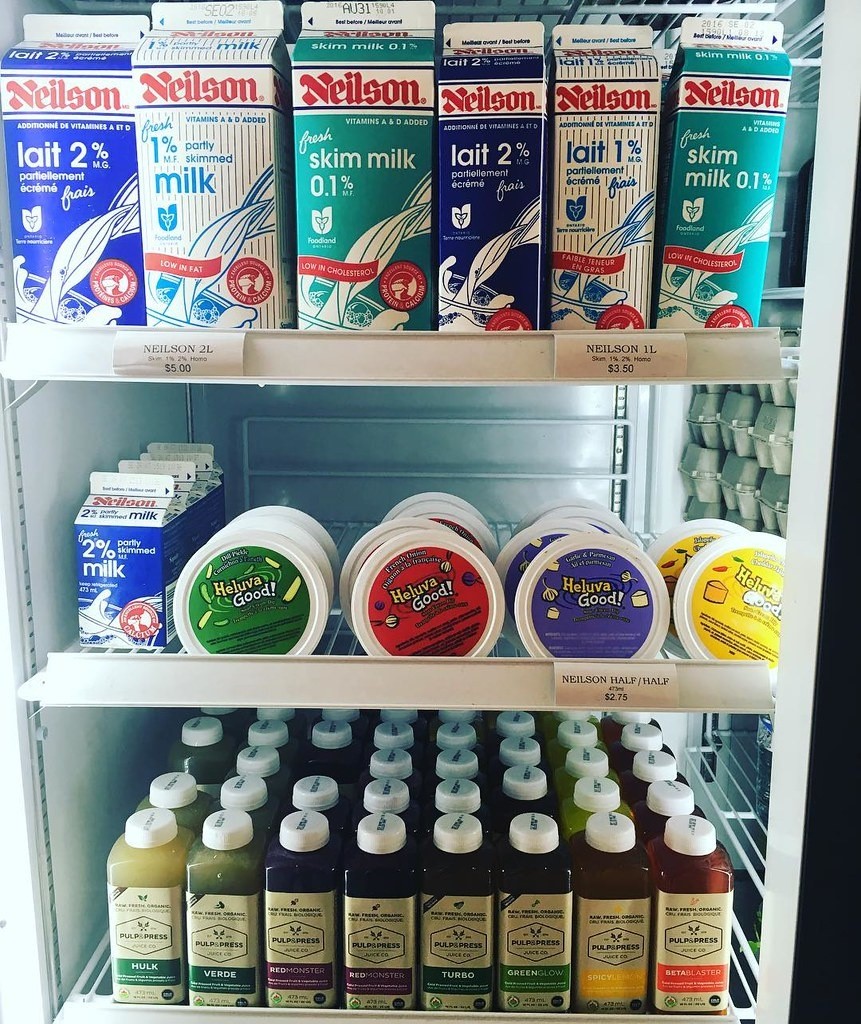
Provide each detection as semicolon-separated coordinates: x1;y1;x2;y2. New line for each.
0;2;861;1024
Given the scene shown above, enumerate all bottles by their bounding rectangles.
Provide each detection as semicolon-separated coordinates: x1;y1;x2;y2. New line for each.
103;707;739;1021
